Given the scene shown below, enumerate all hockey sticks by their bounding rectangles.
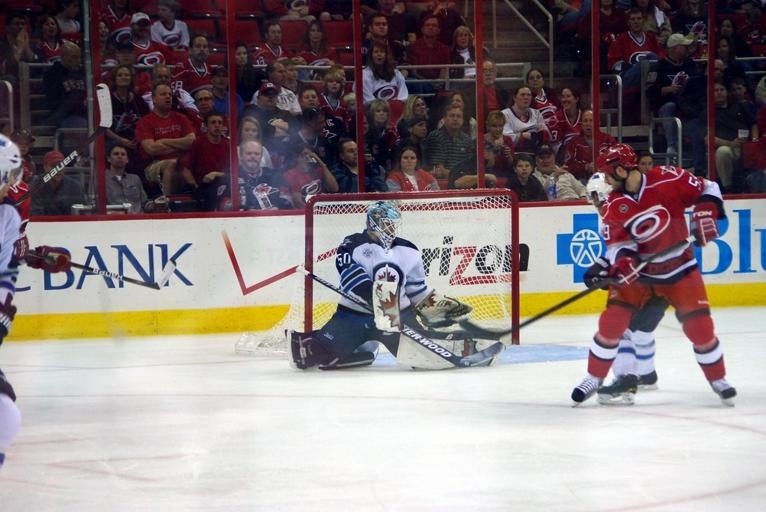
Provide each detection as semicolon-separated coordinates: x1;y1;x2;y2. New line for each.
296;265;505;367
15;83;114;208
459;234;696;341
28;248;176;289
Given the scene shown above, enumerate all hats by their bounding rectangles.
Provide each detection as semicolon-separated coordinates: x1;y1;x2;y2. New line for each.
666;32;694;48
258;81;277;95
535;143;555;156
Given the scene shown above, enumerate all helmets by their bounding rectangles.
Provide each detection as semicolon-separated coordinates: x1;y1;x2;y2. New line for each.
593;142;638;173
586;172;613;202
0;133;23;191
365;199;402;233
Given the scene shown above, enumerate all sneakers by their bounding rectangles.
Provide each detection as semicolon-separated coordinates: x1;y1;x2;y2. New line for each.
597;374;641;397
710;378;737;400
632;369;660;385
571;373;604;402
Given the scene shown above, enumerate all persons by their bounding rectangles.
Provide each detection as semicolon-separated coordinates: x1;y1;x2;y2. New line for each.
282;200;472;371
0;134;29;465
569;143;736;403
586;172;669;396
0;0;766;215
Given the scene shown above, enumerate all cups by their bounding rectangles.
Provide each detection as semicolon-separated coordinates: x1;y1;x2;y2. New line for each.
738;129;750;141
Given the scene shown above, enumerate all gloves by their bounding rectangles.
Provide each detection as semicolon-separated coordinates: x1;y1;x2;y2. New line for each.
583;257;612;291
25;244;72;274
692;201;720;247
610;253;642;285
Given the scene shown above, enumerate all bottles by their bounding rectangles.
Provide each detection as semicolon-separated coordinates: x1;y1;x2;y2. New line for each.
547;177;557;201
